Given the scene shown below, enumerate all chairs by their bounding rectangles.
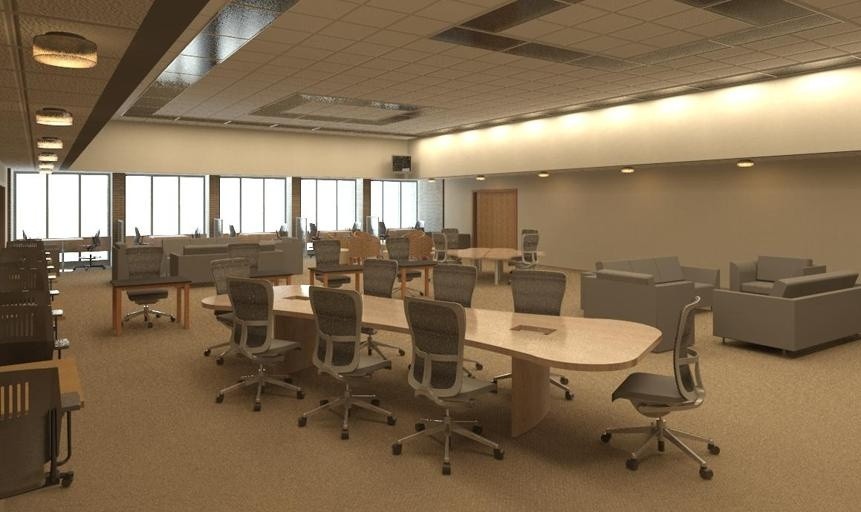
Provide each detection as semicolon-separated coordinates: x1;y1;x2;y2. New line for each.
506;229;539;285
203;256;251;367
0;229;85;502
121;242;178;329
601;295;722;484
70;227;107;272
200;218;460;287
353;258;405;369
432;263;483;378
490;270;575;400
297;284;398;442
215;275;304;413
392;295;505;477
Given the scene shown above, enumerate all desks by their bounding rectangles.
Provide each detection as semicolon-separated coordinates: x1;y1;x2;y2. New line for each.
201;282;665;437
108;275;193;338
444;247;523;285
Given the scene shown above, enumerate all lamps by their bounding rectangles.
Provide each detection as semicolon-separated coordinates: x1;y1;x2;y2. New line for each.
621;167;634;174
736;159;755;168
538;171;549;178
31;29;102;177
427;178;436;183
475;175;485;181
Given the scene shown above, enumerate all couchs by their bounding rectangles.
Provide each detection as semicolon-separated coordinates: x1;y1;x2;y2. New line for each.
596;255;720;309
727;254;826;296
711;268;860;357
113;226;305;291
581;255;696;355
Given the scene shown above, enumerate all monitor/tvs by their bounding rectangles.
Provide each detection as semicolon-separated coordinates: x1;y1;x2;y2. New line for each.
391;154;412;174
